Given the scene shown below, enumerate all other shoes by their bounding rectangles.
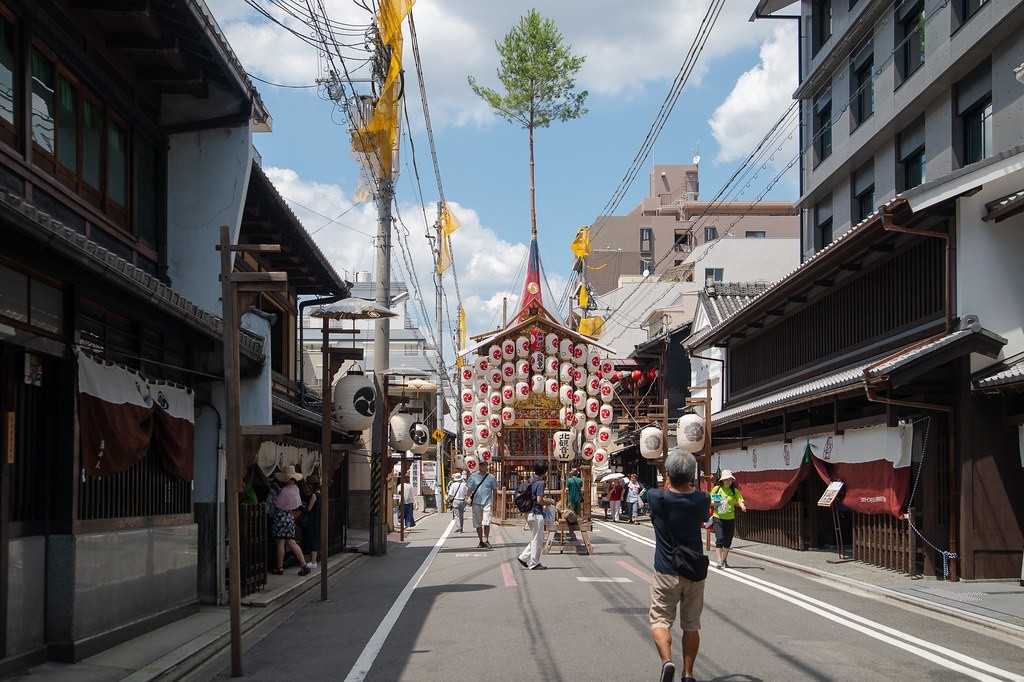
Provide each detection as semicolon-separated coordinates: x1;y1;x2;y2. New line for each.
307;562;317;568
271;567;284;575
660;661;675;682
531;563;547;570
634;522;638;524
454;528;463;533
723;560;729;567
717;561;723;569
298;568;311;576
517;558;528;567
484;541;492;549
477;542;484;548
681;677;696;682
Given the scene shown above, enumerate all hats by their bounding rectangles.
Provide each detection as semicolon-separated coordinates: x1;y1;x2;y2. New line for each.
275;465;303;483
479;460;488;465
719;470;736;482
454;473;463;481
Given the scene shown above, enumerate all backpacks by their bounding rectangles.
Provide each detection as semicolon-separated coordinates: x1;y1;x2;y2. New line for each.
514;478;541;513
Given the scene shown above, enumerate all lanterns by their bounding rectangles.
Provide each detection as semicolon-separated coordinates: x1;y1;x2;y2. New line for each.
677;412;705;452
410;422;429;456
389;409;416;454
640;425;663;460
530;329;559;397
454;335;530;472
559;338;614;466
612;369;657;381
334;370;376;435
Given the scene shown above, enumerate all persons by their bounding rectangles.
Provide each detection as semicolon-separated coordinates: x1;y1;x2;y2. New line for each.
647;450;711;682
465;460;499;548
565;468;583;516
240;464;321;576
710;470;746;568
601;473;645;525
517;460;554;570
447;473;468;532
397;477;416;531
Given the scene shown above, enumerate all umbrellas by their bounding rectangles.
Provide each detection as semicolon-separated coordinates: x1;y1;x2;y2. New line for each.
600;474;625;482
594;473;609;483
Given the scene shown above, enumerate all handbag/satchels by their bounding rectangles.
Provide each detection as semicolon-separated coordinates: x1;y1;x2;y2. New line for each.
638;495;644;508
606;485;616;500
701;507;713;529
470;493;473;506
543;505;555;523
580;495;584;502
449;497;453;503
